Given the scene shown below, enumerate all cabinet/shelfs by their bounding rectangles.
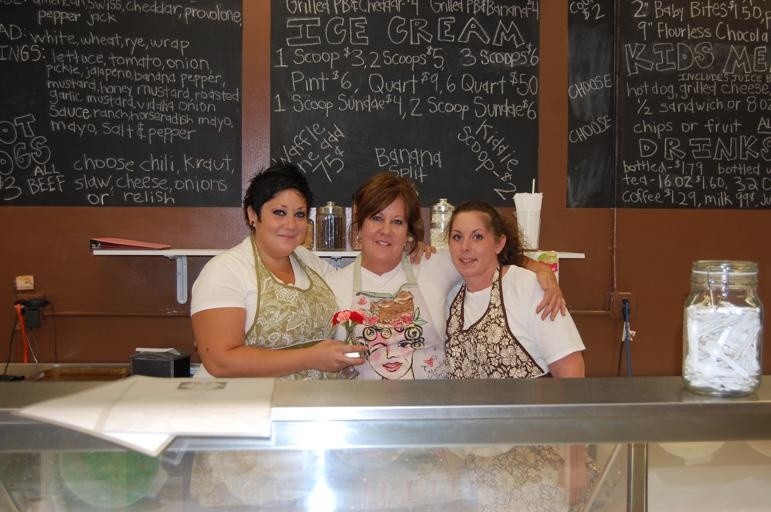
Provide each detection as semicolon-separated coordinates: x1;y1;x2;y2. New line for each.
1;372;770;512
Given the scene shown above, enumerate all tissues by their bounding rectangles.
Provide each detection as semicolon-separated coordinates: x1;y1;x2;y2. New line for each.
131;345;192;380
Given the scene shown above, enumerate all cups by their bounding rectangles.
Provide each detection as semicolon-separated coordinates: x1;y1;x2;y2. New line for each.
515;193;543;252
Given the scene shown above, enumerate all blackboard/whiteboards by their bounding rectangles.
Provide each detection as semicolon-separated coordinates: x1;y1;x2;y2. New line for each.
271;0;540;208
567;0;771;209
0;0;243;207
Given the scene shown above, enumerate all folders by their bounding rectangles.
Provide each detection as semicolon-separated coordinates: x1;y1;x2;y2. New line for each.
88;238;172;250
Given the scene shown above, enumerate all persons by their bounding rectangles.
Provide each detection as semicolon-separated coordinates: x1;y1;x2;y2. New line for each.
446;201;587;512
188;166;436;512
321;174;567;512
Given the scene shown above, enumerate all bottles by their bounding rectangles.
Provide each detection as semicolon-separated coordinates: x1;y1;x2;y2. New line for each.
429;197;457;253
413;189;426;242
301;218;315;250
681;256;766;399
349;192;363;250
317;198;347;251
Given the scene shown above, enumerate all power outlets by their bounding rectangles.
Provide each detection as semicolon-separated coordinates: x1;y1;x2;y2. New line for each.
610;290;635;321
15;291;46;329
